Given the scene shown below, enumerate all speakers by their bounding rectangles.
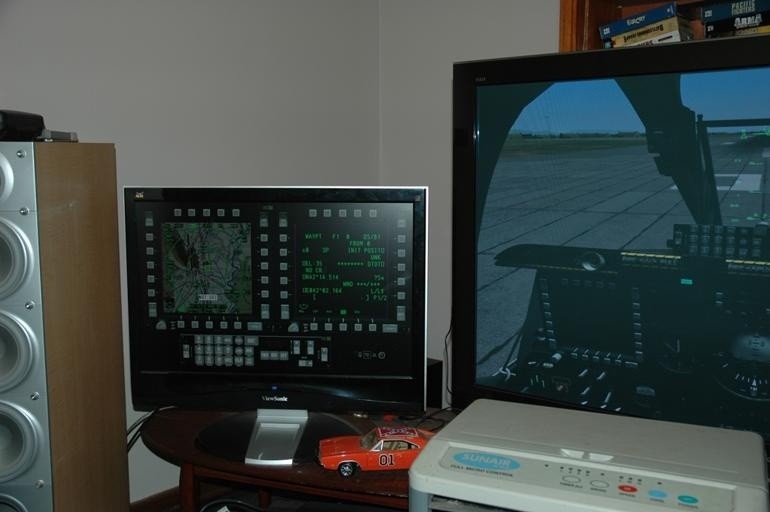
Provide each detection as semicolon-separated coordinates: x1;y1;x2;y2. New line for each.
0;141;130;512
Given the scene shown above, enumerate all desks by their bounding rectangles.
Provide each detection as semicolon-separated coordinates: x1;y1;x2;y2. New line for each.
138;400;463;510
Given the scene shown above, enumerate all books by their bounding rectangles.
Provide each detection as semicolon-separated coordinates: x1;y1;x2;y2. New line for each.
596;0;770;52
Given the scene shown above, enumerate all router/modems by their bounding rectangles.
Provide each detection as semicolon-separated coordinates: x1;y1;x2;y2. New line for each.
372;408;458;435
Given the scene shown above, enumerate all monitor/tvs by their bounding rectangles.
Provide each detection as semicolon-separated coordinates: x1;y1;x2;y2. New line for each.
451;32;770;479
124;185;431;468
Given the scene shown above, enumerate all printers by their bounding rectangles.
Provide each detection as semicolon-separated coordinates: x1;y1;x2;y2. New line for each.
407;398;769;512
